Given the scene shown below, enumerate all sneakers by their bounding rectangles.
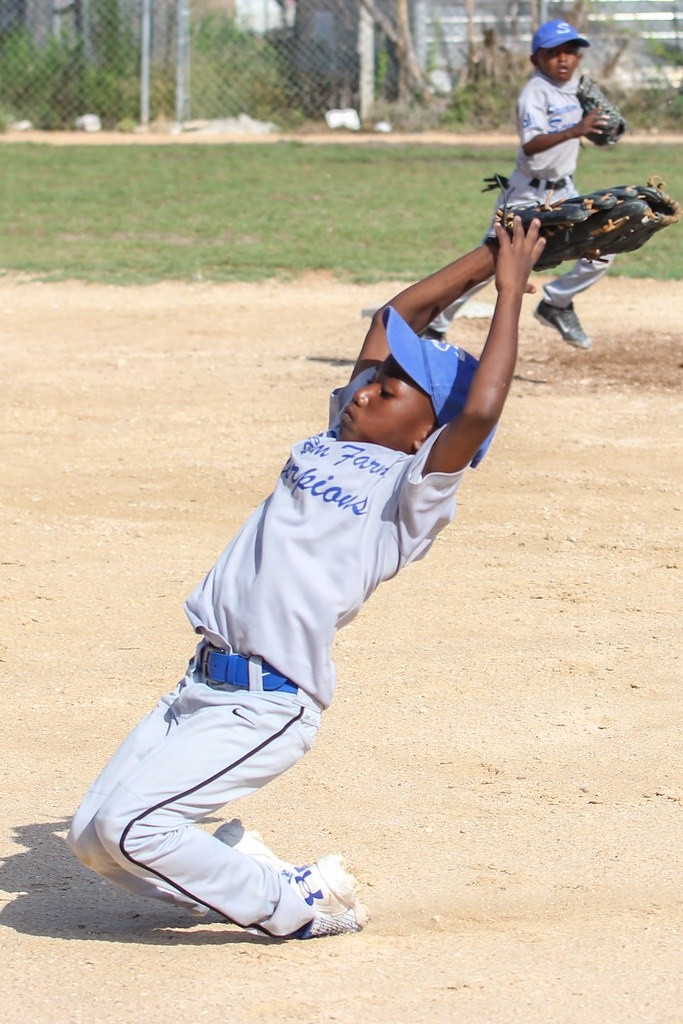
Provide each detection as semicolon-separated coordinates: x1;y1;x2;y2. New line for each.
417;327;447;345
533;297;592;349
290;855;369;940
212;818;300;881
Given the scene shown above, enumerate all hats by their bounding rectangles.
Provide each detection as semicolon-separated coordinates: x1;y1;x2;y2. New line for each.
382;306;498;469
532;20;589;53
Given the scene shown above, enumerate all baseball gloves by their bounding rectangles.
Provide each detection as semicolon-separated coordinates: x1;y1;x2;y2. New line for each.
576;74;627;147
483;183;683;273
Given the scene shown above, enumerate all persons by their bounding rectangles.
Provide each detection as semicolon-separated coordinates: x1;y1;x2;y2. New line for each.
67;175;683;940
417;19;624;350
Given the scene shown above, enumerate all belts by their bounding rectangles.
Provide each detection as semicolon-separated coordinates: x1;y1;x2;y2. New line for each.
529;175;572;191
195;639;298;694
